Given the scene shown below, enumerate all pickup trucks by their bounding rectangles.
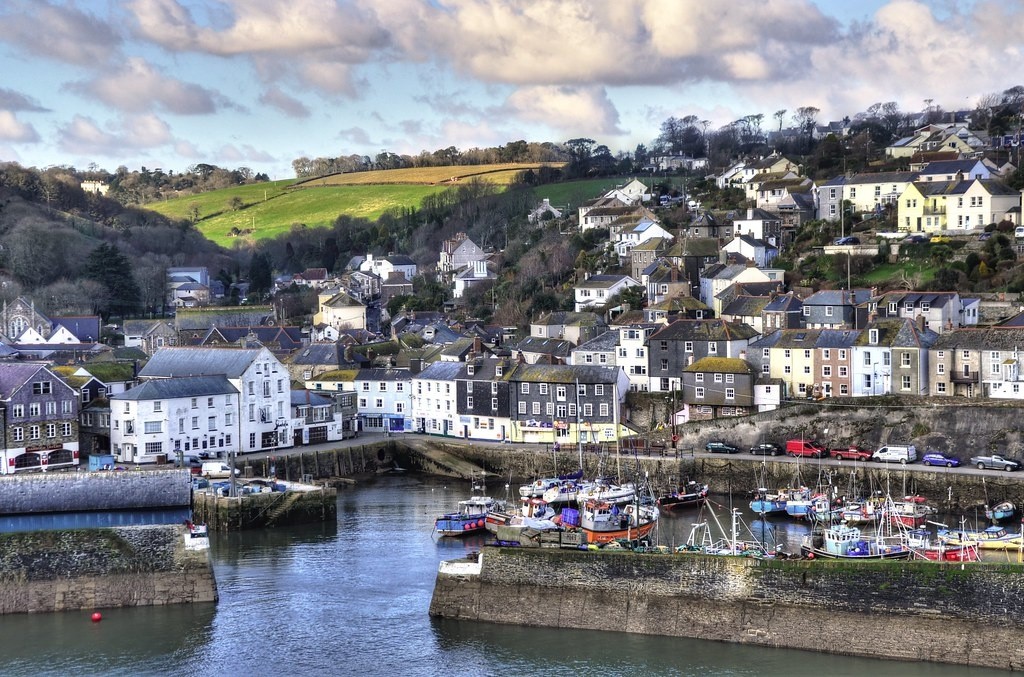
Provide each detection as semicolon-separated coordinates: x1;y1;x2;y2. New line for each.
971;454;1023;472
828;445;873;461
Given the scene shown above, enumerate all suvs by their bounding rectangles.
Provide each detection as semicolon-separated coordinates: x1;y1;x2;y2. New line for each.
704;440;740;455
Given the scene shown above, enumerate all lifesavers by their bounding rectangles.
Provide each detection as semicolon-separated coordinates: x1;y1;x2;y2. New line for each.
630;517;634;525
553;488;558;492
672;435;678;442
588;491;594;496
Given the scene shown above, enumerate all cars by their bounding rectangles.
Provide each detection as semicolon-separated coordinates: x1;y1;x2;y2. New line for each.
922;452;962;468
749;442;783;456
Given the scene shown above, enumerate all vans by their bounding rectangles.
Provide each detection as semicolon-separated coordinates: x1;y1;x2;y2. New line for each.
872;445;918;465
174;455;205;468
787;440;828;458
201;463;241;478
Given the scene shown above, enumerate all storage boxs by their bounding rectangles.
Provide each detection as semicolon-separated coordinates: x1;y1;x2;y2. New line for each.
541;528;589;546
192;479;287;496
847;540;874;556
563;508;580;525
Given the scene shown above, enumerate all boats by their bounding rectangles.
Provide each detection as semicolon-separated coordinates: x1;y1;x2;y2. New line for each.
430;377;1023;569
